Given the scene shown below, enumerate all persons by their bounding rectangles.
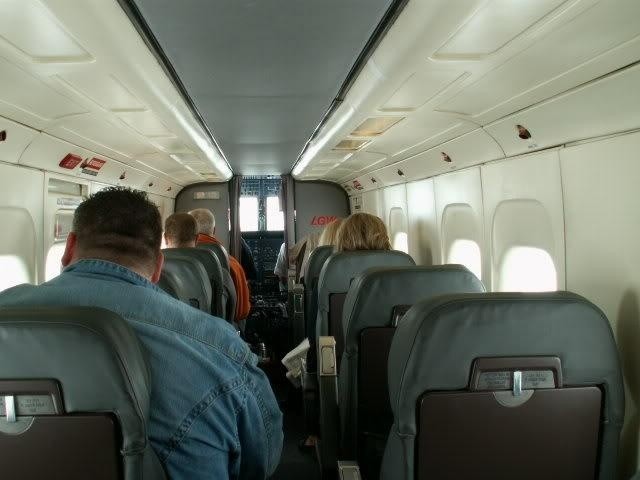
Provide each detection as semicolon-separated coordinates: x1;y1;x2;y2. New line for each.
188;206;251;324
275;239;287;298
165;213;237;336
1;186;283;480
296;214;390;464
317;219;348;247
241;235;256;288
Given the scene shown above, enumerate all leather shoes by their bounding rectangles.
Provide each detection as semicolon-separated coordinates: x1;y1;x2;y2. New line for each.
262;352;277;369
300;438;314;450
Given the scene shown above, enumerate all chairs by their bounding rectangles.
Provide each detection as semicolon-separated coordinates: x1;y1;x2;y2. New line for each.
292;245;333;437
293;240;307;350
300;249;418;455
157;255;212;314
318;264;487;480
156;246;226;320
195;243;231;272
0;306;167;480
338;291;625;480
